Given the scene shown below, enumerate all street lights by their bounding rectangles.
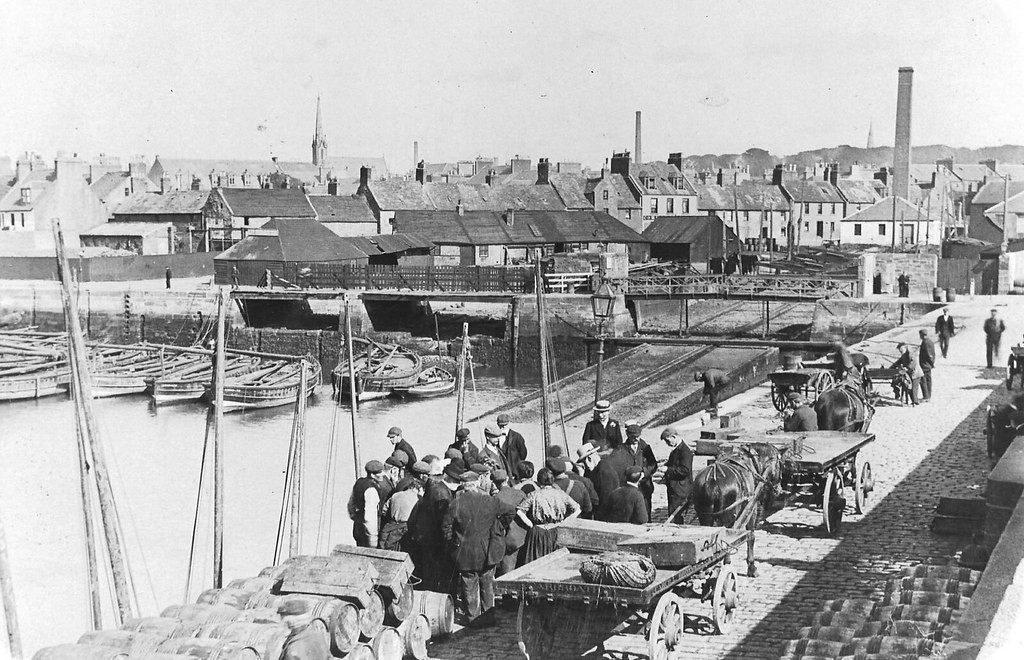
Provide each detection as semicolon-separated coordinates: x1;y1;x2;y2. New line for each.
591;271;617;421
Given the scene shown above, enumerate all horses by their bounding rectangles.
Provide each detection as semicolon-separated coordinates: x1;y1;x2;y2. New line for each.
815;368;866;490
693;443;789;577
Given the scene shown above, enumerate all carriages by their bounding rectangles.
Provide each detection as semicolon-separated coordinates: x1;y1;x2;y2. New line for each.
747;369;878;537
490;442;788;660
984;393;1024;460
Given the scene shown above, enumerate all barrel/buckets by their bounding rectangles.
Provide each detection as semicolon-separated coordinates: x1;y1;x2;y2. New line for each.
783;355;802;370
32;556;454;660
779;565;982;660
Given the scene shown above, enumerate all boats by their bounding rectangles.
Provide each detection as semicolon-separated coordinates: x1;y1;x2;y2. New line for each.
409;366;455;399
203;352;321;415
145;347;261;406
333;342;423;402
0;327;212;398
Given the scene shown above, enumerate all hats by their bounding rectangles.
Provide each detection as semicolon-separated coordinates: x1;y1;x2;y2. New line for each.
548;459;565;474
386;457;402;468
895;341;908;348
576;442;600;463
538;468;554;484
490;469;508;482
627;424;642;434
387;427;403;437
470;464;489;474
592;400;612;412
413;461;431;473
456;428;470;437
422;455;440;465
550;445;562;459
365;460;383;473
788;392;800;399
484;424;501;436
661;428;676;440
448;447;463;459
694;372;700;382
496;414;511;425
442;461;465;480
391;449;408;465
625;467;642;483
461;472;480;481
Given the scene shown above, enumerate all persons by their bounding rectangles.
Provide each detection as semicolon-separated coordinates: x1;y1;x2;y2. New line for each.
919;329;935;402
695;369;731;412
984;309;1005;368
898;271;911;297
889;342;925;405
658;427;693;524
348;400;658;628
936;308;954;358
784;392;818;432
165;266;171;288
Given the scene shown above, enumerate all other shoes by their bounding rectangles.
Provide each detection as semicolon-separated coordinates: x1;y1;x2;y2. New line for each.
910;401;920;405
986;363;992;368
919;398;928;403
